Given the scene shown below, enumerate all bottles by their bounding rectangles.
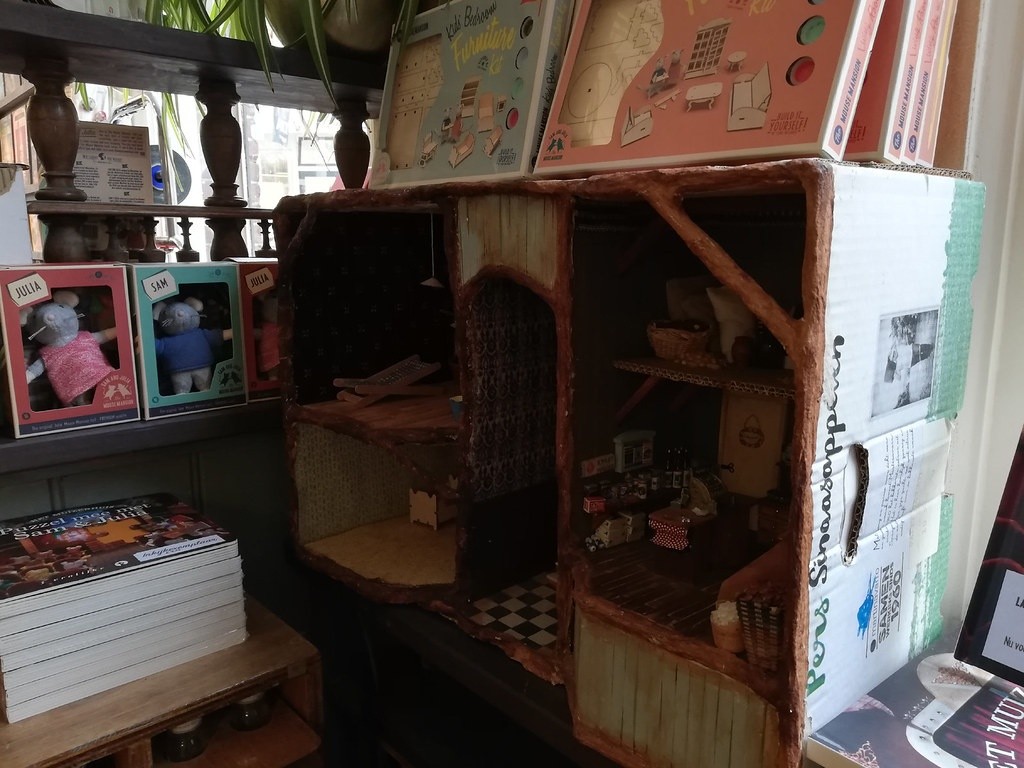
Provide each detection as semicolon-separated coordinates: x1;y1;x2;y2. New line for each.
665;446;691;490
233;694;271;731
164;719;207;762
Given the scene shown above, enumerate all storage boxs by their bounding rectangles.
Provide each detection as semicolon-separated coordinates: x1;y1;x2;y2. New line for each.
0;258;281;439
367;0;959;191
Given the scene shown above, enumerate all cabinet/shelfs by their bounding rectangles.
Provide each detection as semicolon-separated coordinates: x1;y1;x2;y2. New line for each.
272;155;986;768
0;593;328;768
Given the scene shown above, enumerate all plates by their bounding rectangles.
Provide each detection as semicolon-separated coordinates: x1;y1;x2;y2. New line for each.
547;572;557;585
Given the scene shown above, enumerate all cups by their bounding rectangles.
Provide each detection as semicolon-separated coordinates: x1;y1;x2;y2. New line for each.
710;601;744;652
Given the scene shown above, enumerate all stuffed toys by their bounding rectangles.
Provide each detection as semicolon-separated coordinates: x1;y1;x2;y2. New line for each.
18;291;117;406
153;297;233;395
254;291;280;380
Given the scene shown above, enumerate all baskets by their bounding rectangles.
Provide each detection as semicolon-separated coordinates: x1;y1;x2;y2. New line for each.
647;317;715;360
739;596;786;672
